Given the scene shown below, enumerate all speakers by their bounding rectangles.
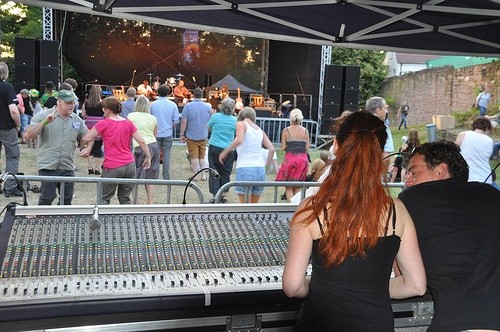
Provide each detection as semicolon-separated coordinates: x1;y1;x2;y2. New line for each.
320;64;359;148
13;37;59;96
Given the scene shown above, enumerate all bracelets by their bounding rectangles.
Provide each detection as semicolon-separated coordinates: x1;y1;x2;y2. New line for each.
42;120;49;126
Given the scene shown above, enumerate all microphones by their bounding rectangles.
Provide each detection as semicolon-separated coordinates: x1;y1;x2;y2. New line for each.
90;205;101;230
181;168;220;204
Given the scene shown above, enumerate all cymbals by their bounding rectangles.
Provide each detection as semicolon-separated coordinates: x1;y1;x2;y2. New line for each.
154;77;162;79
147;74;154;75
176;73;184;76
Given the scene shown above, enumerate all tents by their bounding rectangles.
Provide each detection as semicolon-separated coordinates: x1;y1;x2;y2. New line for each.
206;74;261;99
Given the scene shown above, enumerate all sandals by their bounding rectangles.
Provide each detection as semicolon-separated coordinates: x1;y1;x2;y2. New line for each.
219;194;228;203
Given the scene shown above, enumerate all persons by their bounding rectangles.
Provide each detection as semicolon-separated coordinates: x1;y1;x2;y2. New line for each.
394;139;500;332
475;85;490;117
486;106;500;126
455;116;493;184
282;111;426;332
0;62;420;207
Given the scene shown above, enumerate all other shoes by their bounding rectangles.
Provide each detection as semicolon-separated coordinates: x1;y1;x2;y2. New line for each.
4;189;24;198
187;176;198;181
201;176;207;181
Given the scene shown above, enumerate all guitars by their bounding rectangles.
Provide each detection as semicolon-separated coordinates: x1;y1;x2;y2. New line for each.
235;87;243;110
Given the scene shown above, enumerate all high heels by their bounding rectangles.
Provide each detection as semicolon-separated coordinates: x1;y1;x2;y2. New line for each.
94;167;100;175
87;166;94;175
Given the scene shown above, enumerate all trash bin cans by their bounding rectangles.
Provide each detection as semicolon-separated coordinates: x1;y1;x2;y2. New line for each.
426;123;437;143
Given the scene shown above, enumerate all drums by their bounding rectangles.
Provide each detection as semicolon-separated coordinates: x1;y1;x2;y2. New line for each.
166;78;177;87
152;81;161;89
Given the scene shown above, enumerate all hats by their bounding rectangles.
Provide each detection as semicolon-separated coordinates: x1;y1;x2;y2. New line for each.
45;81;55;87
290;108;304;122
193;88;203;95
20;89;30;94
58;89;79;103
